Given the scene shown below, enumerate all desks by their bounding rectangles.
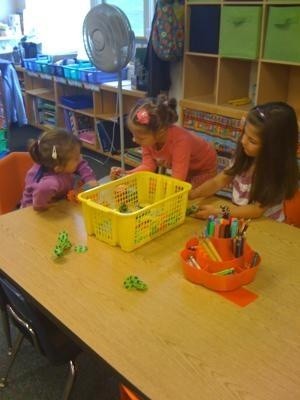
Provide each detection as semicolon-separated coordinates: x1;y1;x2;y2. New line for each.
0;173;300;400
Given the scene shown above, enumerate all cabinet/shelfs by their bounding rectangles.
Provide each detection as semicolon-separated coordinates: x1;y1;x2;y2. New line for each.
178;1;299;202
15;66;169;168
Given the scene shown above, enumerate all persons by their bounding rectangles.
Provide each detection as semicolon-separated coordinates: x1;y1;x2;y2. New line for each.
20;127;97;210
188;101;297;220
111;94;217;189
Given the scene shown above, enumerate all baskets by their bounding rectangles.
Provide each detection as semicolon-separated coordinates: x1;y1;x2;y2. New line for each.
22;55;128;83
78;171;192;254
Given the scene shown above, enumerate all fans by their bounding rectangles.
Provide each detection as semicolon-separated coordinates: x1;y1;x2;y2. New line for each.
83;3;136;176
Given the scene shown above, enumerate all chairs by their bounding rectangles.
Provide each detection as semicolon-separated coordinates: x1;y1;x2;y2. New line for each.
0;150;88;399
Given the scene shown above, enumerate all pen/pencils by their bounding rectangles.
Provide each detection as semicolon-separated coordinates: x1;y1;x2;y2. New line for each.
186;214;258;275
